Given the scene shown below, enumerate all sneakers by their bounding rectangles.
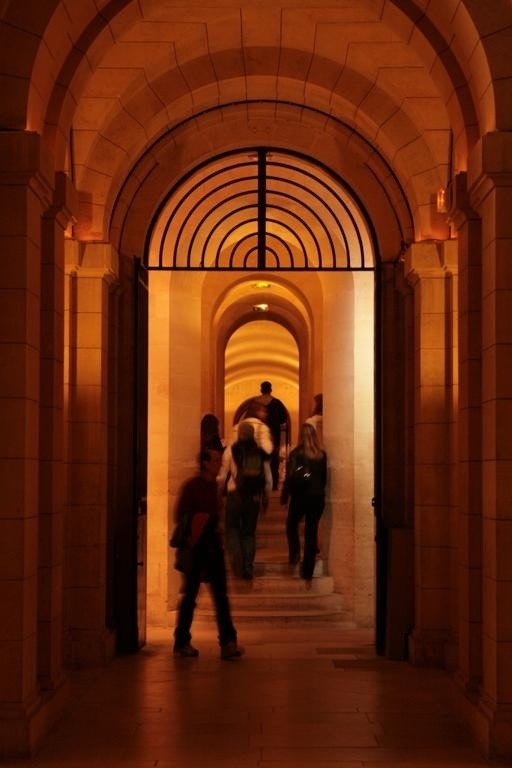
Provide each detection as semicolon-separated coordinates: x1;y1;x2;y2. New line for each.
172;641;198;657
222;643;243;659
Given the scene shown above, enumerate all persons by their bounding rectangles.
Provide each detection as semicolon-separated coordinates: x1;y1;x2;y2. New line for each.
200;413;226;454
242;381;289;491
277;423;328;584
170;442;246;662
304;393;324;449
213;422;273;581
